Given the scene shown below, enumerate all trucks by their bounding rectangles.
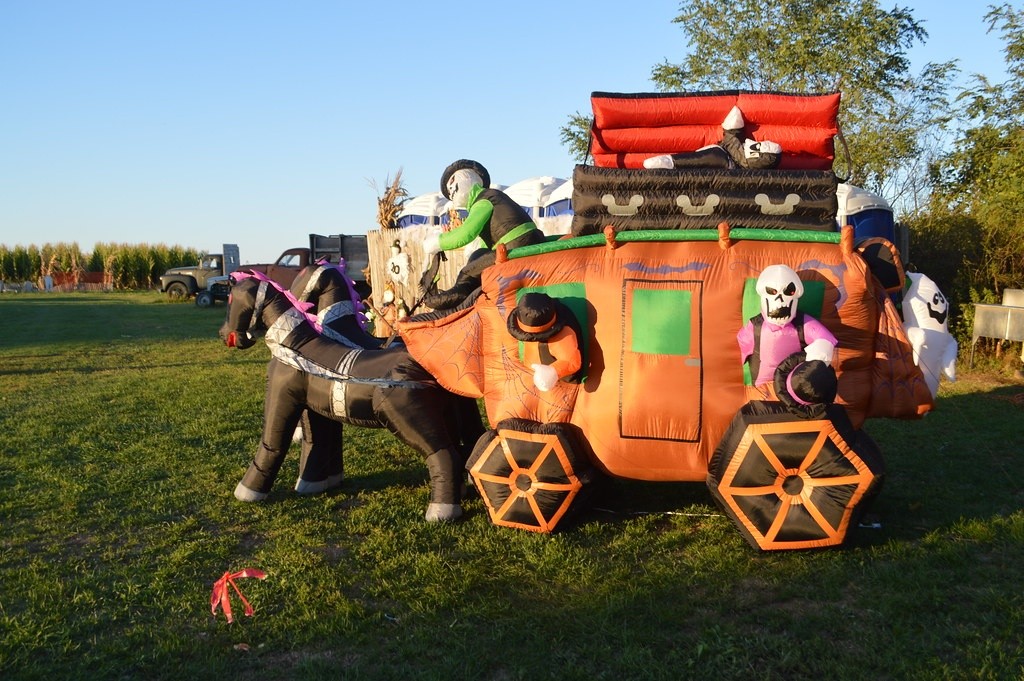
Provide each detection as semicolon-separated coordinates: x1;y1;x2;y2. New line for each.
159;254;273;301
239;233;368;292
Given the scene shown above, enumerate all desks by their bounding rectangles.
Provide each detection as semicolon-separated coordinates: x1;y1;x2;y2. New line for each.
970;288;1024;371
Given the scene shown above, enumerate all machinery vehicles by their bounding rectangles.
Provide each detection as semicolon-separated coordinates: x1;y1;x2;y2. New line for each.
195;273;233;307
